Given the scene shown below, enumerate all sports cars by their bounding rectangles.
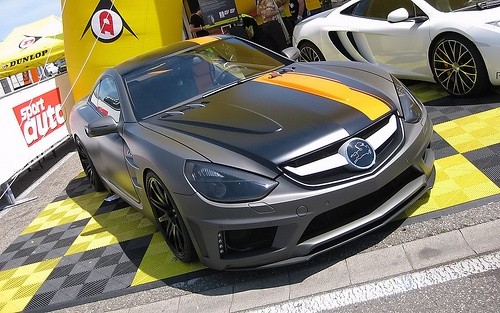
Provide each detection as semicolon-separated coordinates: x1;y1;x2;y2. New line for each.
292;1;500;100
66;32;434;271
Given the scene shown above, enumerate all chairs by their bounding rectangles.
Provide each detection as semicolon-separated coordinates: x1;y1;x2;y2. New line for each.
131;85;162;114
186;57;219;98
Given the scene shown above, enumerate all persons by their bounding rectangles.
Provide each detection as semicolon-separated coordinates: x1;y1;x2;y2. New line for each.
319;0;333;12
189;8;209;38
232;12;259;45
290;0;307;27
257;0;288;54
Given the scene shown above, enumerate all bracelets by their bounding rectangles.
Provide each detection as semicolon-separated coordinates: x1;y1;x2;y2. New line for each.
278;8;281;14
